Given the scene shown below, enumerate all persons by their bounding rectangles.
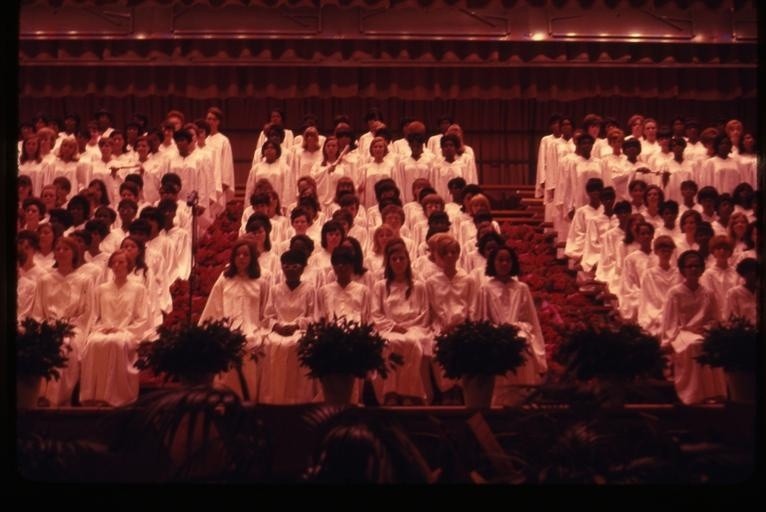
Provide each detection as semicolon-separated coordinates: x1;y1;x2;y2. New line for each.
17;104;235;406
198;106;548;406
535;111;760;407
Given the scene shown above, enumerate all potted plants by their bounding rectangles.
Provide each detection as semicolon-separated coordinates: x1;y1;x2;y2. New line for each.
15;311;79;406
551;318;670;407
290;313;403;404
135;313;253;390
433;317;528;407
693;311;765;402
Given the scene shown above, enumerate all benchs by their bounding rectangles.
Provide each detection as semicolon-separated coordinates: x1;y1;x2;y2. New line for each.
135;180;672;385
